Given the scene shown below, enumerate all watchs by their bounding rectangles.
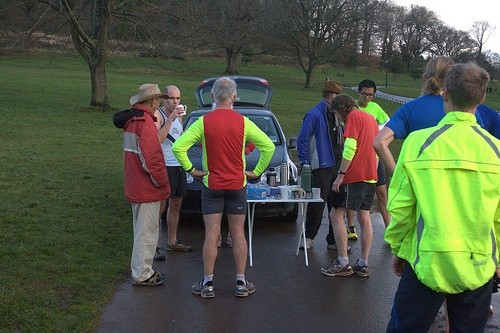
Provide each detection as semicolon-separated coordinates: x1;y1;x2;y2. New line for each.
337;169;346;175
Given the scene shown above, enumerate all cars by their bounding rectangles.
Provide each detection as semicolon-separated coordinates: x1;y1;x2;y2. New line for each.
161;76;300;222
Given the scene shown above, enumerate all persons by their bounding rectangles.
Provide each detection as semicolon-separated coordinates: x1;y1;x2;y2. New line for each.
173;55;500;333
114;84;170;286
153;86;194;261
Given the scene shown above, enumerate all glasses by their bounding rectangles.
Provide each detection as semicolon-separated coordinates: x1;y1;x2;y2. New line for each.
361;92;374;98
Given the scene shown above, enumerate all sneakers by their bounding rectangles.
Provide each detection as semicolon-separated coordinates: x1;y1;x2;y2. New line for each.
354;259;370;277
235;280;256;297
132;271;169;287
192;280;216;298
166;241;193;252
154;248;166;261
321;258;354;277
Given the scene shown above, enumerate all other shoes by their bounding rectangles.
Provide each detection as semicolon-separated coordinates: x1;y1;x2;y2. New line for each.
327;242;352;252
347;226;359;240
299;238;314;250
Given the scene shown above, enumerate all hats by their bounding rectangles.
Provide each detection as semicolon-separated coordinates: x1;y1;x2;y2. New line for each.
322;80;342;94
129;84;169;106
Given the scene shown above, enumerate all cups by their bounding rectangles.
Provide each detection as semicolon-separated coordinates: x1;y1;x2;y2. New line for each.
292;188;306;199
280;187;291;199
275;193;281;200
175;104;187;116
312;188;320;199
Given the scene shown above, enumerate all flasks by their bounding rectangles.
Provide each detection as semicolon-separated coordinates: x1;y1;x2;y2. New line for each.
266;167;277;187
280;162;288;185
301;164;312;198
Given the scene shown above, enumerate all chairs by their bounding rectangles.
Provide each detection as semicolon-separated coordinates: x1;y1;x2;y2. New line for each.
255;120;266;132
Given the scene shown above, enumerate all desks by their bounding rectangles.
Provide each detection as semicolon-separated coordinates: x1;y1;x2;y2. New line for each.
246;197;325;267
